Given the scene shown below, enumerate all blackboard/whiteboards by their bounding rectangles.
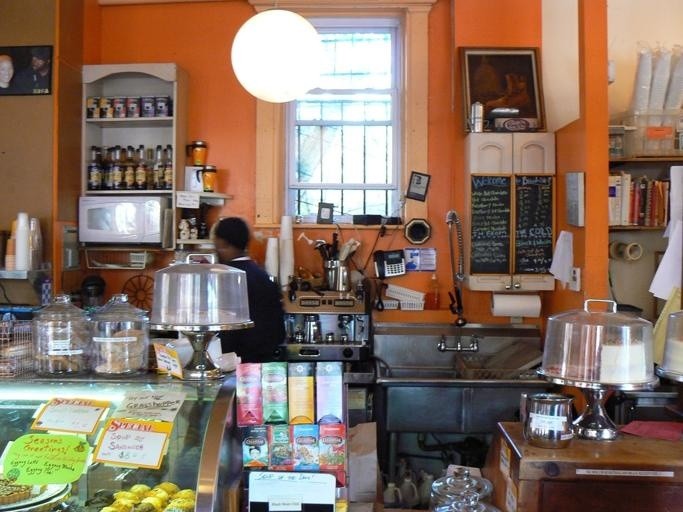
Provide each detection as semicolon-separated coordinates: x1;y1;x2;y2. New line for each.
515;175;552;274
471;176;511;274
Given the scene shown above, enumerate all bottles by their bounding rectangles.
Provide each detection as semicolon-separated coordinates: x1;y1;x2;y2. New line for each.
87;143;172;190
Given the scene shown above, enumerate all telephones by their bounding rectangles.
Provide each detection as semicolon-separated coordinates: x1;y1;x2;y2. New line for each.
374;249;406;312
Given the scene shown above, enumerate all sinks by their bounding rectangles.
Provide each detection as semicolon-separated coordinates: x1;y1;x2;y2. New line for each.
377;363;550;384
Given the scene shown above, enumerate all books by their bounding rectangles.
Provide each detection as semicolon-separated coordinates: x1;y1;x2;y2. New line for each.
608;172;669;228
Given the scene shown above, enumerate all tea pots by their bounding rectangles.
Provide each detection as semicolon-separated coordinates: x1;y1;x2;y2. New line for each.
322;258;351;292
465;99;490;133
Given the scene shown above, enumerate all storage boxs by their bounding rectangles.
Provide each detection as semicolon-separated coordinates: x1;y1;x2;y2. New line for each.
608;125;626;158
609;107;683;157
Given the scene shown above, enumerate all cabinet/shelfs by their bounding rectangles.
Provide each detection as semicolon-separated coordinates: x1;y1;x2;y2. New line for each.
496;419;683;512
176;192;233;246
1;368;245;511
80;62;188;252
608;155;682;232
462;133;556;293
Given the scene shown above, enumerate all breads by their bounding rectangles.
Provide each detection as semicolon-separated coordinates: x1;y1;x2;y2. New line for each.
97;326;146;374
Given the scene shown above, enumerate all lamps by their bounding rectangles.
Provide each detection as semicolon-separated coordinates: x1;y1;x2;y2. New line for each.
230;0;326;103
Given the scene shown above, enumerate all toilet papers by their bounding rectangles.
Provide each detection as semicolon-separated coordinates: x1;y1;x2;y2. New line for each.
489;293;542;320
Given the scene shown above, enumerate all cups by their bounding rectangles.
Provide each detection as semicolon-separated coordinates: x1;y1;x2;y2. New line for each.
0;210;44;272
519;390;575;449
184;140;207;167
195;165;216;193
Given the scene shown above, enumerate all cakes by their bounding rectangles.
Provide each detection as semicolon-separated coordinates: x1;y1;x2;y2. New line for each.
76;481;195;512
0;480;33;505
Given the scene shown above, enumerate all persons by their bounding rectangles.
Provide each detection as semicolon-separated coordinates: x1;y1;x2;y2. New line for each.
0;54;22;94
405;257;417;271
247;446;266;468
212;218;287;361
13;48;50;93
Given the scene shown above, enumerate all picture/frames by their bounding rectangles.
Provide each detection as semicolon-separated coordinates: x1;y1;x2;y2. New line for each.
317;203;333;225
404;170;431;202
459;46;547;133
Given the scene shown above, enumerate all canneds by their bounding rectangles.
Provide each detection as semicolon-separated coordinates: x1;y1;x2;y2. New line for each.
192;140;207;166
203;165;217;192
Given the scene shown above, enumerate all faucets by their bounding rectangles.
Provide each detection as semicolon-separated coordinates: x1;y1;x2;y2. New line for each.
453;317;467;352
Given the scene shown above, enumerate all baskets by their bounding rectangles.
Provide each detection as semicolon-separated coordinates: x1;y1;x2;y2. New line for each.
375;299;398;310
401;301;425;311
0;320;34;377
